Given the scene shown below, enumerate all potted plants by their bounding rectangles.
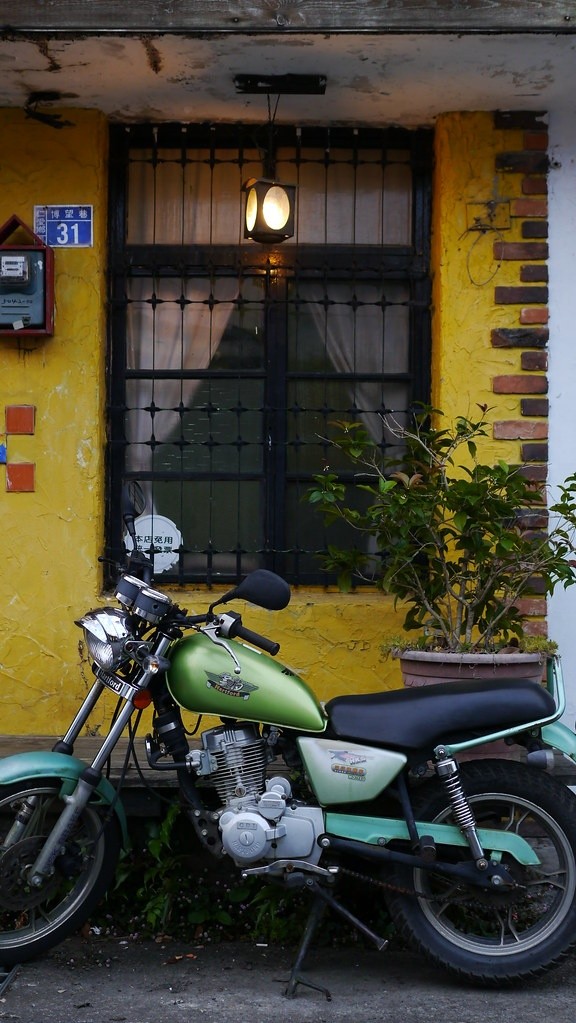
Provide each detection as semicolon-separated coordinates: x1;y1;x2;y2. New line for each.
301;402;576;760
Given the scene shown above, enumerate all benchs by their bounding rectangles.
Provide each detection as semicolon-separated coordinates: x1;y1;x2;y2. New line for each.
0;737;576;872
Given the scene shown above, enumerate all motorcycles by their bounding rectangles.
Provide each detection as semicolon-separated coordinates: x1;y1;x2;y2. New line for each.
0;479;576;1001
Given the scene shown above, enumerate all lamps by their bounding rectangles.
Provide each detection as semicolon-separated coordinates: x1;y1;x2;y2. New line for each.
241;90;299;244
